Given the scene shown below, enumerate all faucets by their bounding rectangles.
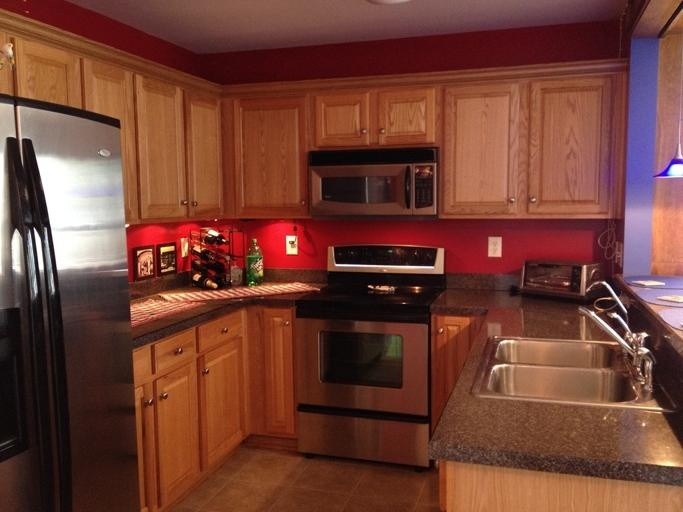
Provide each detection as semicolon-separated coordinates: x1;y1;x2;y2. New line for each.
577;279;657;400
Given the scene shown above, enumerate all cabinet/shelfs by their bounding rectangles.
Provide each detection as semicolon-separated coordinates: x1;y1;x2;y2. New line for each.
0;8;9;95
196;308;248;485
438;58;629;219
245;304;298;452
430;313;487;439
7;11;140;225
132;327;201;512
223;80;311;220
134;54;223;224
310;72;440;152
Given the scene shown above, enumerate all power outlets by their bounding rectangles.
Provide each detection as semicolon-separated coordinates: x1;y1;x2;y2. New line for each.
286;235;298;256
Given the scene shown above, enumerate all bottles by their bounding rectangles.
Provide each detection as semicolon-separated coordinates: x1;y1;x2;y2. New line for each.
190;226;230;290
246;239;264;286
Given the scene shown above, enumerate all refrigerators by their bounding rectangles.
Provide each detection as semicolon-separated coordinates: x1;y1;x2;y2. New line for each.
0;92;142;512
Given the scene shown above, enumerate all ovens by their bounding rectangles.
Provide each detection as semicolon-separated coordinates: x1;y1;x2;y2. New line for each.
292;306;433;475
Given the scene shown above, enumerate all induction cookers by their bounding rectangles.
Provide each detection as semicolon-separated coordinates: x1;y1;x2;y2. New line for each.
296;272;444;312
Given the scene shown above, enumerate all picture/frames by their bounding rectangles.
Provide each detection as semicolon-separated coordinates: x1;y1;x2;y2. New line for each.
133;244;156;281
156;241;177;277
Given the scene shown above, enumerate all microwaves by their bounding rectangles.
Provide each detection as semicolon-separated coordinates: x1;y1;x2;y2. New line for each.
307;146;438;217
522;258;606;302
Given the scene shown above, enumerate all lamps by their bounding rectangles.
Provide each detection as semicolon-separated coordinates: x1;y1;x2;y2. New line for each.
653;76;683;179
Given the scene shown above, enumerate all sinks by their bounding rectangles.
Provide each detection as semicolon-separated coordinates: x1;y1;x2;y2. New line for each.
469;364;677;413
485;333;633;369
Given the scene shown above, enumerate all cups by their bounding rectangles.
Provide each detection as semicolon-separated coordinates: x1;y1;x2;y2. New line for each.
231;269;242;286
